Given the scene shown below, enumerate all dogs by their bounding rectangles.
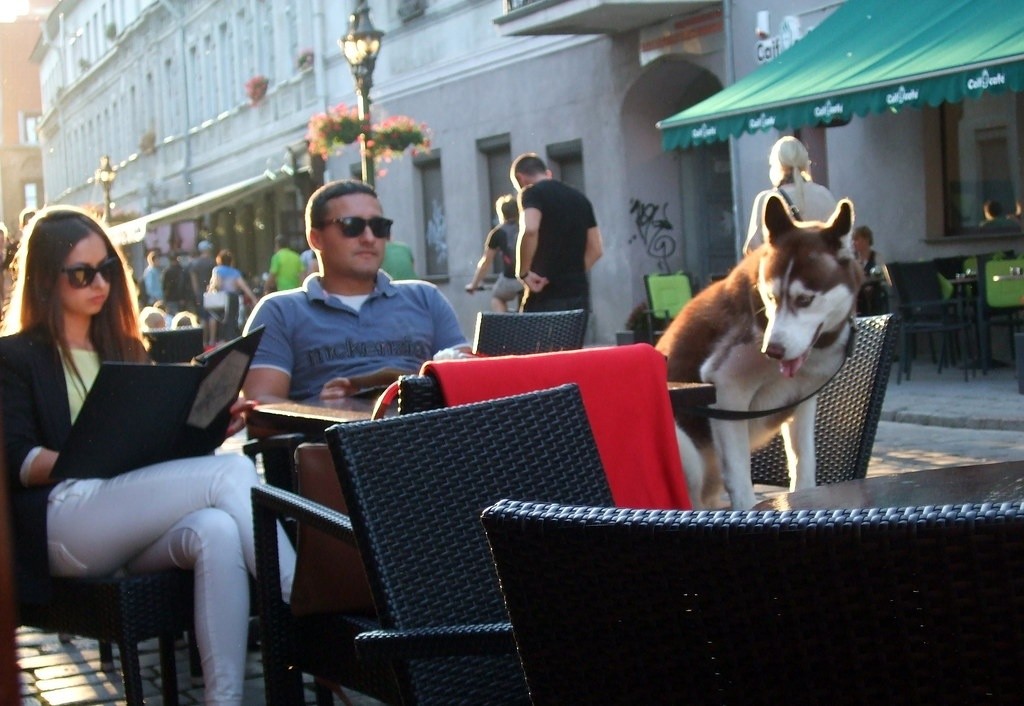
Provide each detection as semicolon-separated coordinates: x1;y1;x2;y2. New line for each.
652;194;865;509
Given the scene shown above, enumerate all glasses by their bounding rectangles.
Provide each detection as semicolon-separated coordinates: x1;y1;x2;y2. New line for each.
60;258;120;288
318;217;393;239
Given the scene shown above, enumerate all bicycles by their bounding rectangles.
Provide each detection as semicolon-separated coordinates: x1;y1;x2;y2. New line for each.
467;284;522;313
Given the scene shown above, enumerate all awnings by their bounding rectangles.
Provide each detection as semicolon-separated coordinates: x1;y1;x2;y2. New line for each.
655;0;1024;150
108;166;289;245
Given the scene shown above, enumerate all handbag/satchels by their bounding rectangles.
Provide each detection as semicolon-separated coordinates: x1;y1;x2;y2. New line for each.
291;379;379;615
203;291;229;324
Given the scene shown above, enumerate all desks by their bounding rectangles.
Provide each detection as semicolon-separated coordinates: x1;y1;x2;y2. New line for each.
246;381;714;510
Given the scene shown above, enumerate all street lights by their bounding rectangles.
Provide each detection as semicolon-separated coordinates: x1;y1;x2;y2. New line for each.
337;0;385;188
95;155;118;229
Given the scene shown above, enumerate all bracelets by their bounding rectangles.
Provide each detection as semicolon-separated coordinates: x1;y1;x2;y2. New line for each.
515;273;528;279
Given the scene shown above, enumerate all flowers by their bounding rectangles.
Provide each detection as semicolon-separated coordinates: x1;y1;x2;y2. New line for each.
293;48;315;71
304;103;435;174
243;75;267;107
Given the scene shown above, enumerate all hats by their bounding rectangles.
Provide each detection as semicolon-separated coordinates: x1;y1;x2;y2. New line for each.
198;241;214;252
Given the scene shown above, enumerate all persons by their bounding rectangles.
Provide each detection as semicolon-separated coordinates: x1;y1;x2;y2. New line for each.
0;212;36;320
852;226;893;286
380;241;417;280
262;233;319;295
982;200;1014;226
0;211;297;706
137;241;258;348
468;196;527;313
743;136;836;254
242;178;472;438
510;153;603;313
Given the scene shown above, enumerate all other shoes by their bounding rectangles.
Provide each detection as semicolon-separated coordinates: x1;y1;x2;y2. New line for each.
204;345;209;350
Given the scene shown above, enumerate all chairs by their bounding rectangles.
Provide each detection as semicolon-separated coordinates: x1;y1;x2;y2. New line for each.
14;258;1023;706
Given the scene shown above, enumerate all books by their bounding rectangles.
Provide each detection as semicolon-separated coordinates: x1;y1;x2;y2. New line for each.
47;325;266;481
